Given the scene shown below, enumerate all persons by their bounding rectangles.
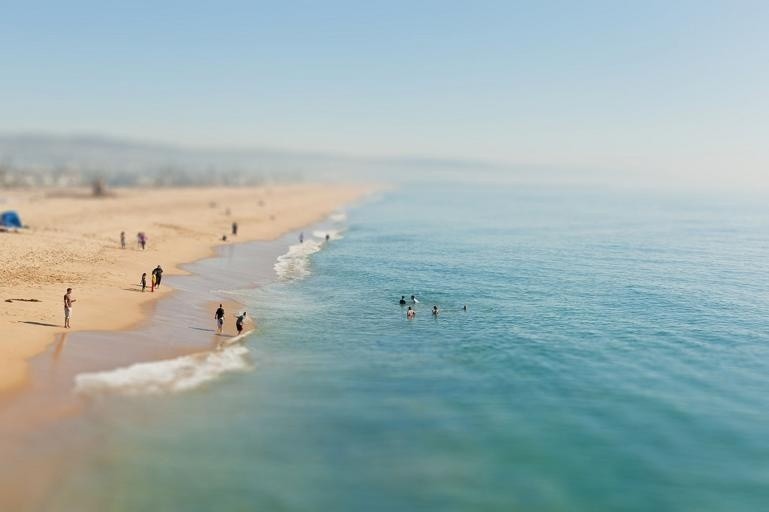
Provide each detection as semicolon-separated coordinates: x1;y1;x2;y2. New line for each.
235;311;246;335
63;288;76;328
215;303;224;332
121;222;329;292
400;296;467;319
